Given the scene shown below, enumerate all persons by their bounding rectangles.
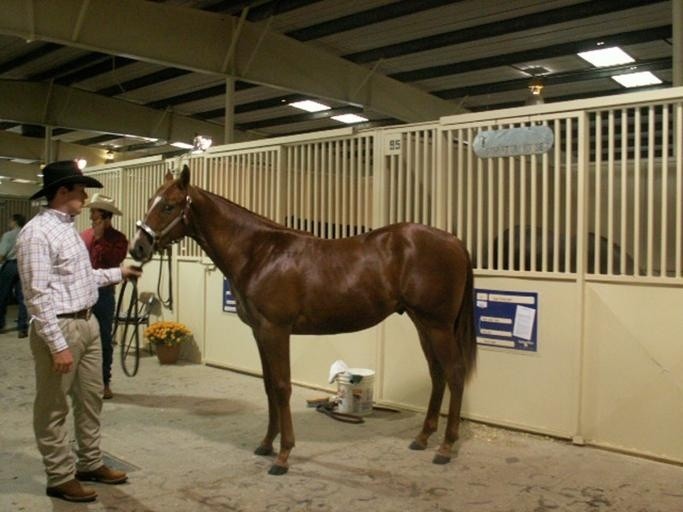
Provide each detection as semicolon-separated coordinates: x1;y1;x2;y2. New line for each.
15;157;142;503
78;191;129;399
0;213;31;338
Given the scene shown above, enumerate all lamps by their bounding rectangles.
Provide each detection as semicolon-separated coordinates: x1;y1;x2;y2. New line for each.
105;149;114;163
526;75;543;105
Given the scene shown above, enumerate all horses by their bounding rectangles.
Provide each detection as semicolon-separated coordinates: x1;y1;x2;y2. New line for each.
129;163;477;475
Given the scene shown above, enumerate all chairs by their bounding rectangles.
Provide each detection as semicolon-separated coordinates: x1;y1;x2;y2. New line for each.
112;292;155;360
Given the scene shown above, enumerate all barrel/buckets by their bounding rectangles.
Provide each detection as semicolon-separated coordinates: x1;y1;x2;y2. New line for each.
335;370;375;416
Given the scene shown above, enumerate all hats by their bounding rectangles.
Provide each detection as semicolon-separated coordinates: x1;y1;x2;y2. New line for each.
82;193;123;218
29;160;103;201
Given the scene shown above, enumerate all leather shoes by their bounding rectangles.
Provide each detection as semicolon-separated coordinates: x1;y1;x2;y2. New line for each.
46;479;98;501
75;465;128;484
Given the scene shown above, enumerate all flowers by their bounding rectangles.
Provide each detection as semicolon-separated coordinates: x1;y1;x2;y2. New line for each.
144;321;194;344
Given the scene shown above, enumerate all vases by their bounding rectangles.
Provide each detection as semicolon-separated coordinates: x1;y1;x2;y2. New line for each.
156;343;181;365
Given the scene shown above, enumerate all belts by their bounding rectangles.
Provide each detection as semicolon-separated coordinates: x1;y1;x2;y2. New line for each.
57;308;92;321
